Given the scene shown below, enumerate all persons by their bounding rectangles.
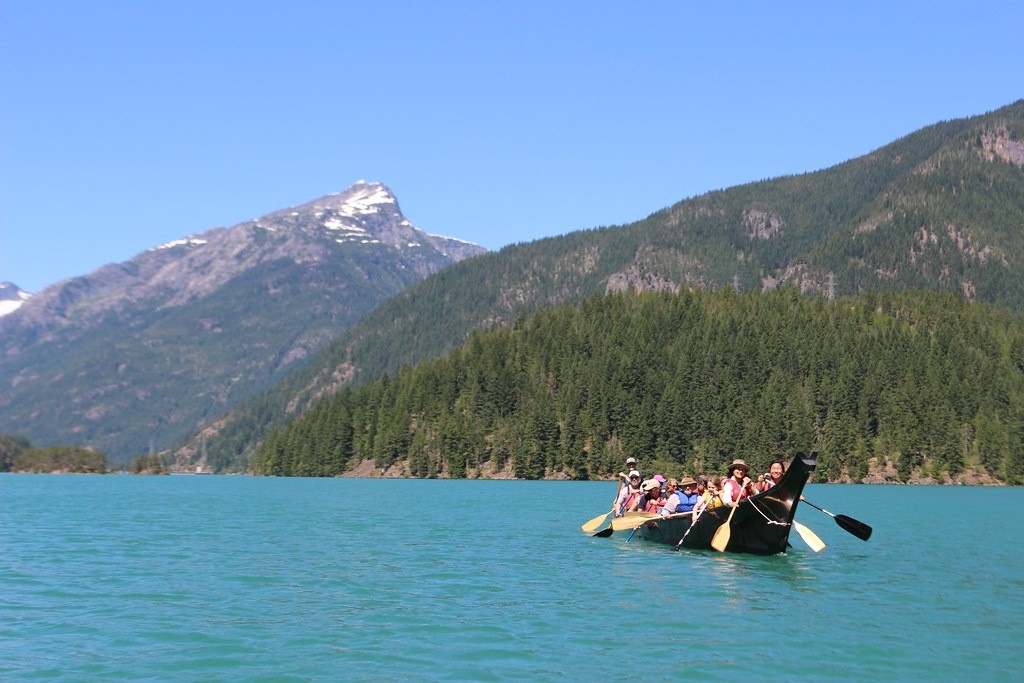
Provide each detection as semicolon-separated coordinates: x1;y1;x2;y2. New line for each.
612;457;805;525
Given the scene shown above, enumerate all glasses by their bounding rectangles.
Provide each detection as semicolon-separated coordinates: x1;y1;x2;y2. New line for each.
671;485;677;487
630;476;639;480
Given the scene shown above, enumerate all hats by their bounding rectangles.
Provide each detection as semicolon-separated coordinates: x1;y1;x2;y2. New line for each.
653;475;667;482
627;457;636;466
677;475;697;489
643;479;660;491
629;471;640;478
727;459;750;472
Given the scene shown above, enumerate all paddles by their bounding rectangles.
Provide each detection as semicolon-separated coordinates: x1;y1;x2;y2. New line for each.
764;479;872;541
626;503;652;542
670;493;714;552
614;476;623;503
592;492;633;538
793;519;826;553
612;509;701;531
581;508;615;532
710;477;749;553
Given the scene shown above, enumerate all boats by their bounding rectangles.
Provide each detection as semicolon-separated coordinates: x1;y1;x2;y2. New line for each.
626;450;820;558
